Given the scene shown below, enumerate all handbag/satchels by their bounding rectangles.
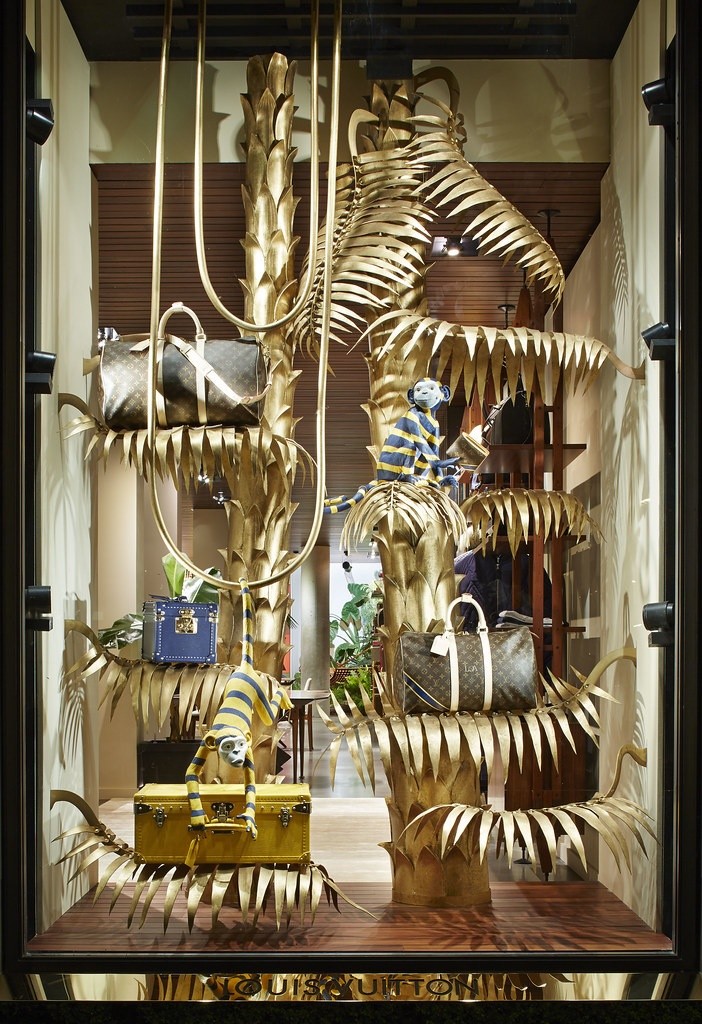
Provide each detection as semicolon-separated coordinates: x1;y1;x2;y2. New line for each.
394;594;537;716
97;302;272;431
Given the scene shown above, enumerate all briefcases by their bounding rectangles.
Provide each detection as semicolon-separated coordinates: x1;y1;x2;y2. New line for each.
142;595;218;665
133;783;311;864
